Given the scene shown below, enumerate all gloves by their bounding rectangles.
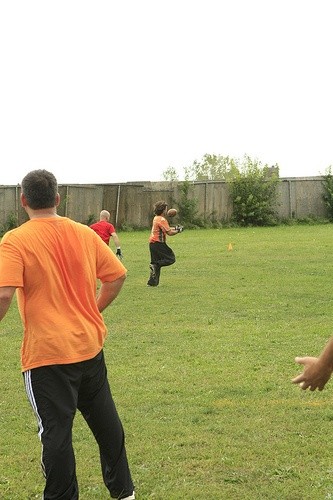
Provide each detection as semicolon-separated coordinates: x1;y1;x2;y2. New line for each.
175;224;181;229
116;249;121;257
177;226;183;232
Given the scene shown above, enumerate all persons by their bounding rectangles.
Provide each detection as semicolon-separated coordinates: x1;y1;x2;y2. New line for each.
287;327;333;394
89;208;125;261
146;197;183;288
0;167;139;500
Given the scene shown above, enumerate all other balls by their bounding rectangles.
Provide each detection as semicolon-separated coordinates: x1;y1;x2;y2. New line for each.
168;209;176;217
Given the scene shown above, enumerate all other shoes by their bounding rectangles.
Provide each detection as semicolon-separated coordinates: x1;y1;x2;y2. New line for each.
148;263;156;276
119;491;135;500
